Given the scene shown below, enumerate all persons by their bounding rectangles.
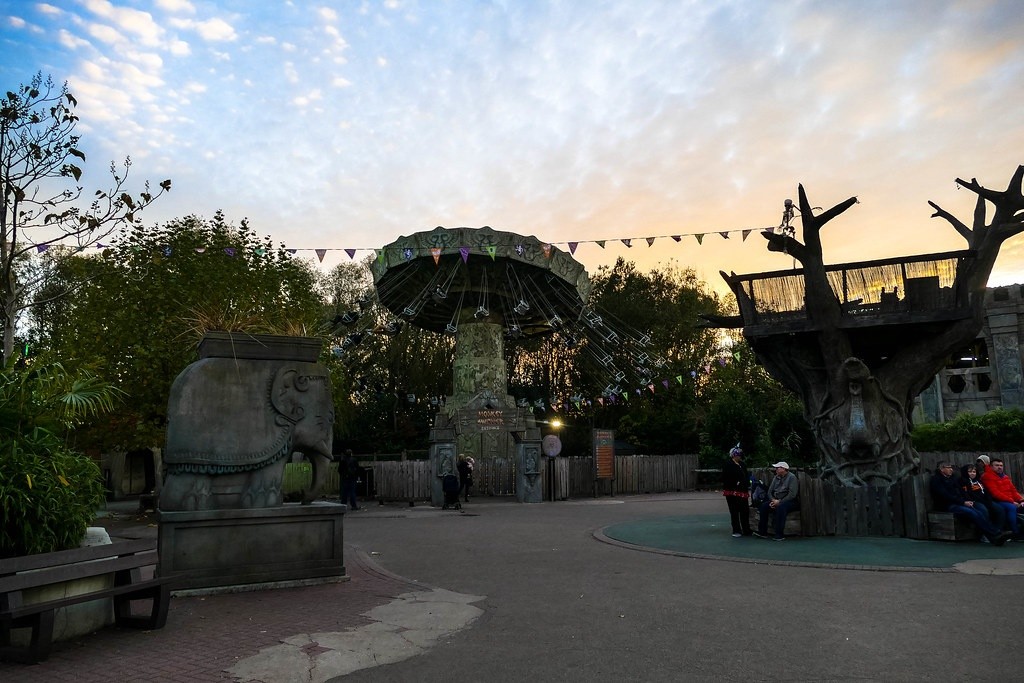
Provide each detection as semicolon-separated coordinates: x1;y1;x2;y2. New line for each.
753;461;799;541
722;445;758;538
337;448;362;510
981;458;1024;542
958;464;1008;543
456;454;473;503
975;455;991;480
466;455;475;478
929;460;1014;546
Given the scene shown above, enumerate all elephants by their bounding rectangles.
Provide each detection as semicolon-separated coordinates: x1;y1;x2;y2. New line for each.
158;358;335;510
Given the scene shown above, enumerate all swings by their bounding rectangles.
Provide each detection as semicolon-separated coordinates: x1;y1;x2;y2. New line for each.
329;259;669;405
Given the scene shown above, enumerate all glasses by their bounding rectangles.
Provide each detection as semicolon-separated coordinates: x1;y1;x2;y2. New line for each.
945;467;953;469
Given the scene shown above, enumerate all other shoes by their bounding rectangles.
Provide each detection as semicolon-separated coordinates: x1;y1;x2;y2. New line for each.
981;530;1024;544
732;533;742;537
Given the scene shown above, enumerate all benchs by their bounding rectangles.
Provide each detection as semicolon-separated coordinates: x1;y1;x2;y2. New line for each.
736;504;801;537
0;536;191;666
925;506;1024;543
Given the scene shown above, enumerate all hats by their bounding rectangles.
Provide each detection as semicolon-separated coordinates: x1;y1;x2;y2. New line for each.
771;461;790;469
977;454;991;467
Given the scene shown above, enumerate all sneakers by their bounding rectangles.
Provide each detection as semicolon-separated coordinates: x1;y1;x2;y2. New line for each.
754;532;768;539
771;536;786;541
